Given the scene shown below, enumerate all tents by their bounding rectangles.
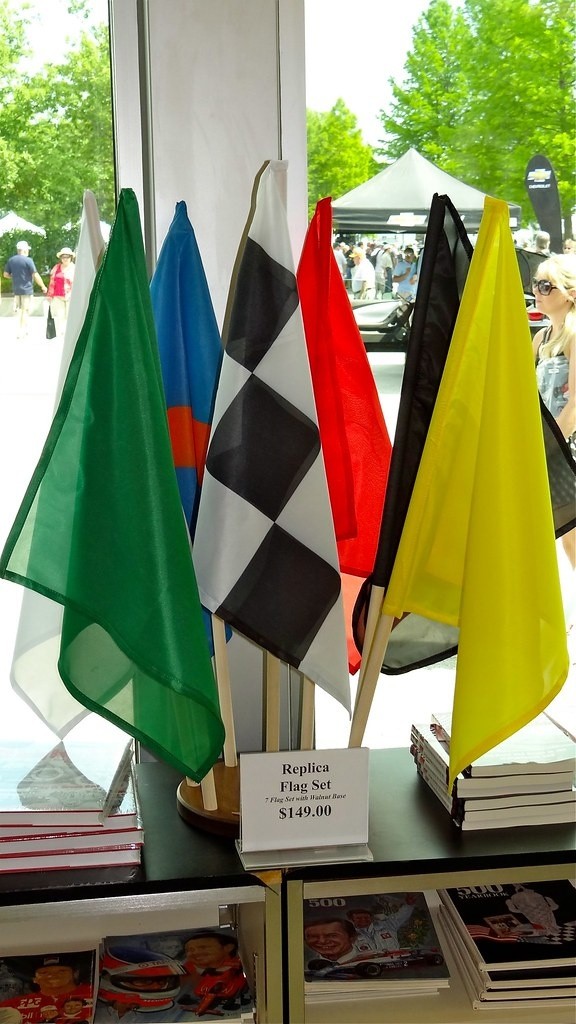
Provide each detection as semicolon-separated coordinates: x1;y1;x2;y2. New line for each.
332;148;521;236
0;213;47;238
62;217;110;243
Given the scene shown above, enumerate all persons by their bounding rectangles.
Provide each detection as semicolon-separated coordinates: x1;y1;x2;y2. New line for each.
48;247;75;322
4;240;47;336
562;239;576;256
535;231;551;257
332;241;424;300
532;254;576;570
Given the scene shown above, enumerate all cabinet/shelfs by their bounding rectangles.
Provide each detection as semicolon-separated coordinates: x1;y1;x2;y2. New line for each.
0;745;576;1023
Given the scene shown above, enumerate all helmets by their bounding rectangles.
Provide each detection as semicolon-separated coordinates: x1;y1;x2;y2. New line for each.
100;944;186;1011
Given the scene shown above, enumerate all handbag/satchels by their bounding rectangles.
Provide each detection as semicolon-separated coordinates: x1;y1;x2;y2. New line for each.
46;305;57;339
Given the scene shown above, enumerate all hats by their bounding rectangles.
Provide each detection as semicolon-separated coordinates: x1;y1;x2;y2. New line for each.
34;956;74;974
383;244;392;250
16;240;31;251
56;247;73;258
349;247;364;257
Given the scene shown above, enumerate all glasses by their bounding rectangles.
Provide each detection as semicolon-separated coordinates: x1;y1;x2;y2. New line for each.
60;254;70;258
531;277;569;296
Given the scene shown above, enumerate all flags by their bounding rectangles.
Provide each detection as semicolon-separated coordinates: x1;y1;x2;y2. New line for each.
350;193;576;678
386;193;570;797
1;158;357;784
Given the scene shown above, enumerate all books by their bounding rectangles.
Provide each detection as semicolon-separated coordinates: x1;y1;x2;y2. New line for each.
1;739;144;875
436;880;576;1010
410;711;576;832
303;888;451;1006
0;926;256;1024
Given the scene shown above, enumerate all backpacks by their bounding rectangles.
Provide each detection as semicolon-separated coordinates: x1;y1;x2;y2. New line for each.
368;249;380;269
